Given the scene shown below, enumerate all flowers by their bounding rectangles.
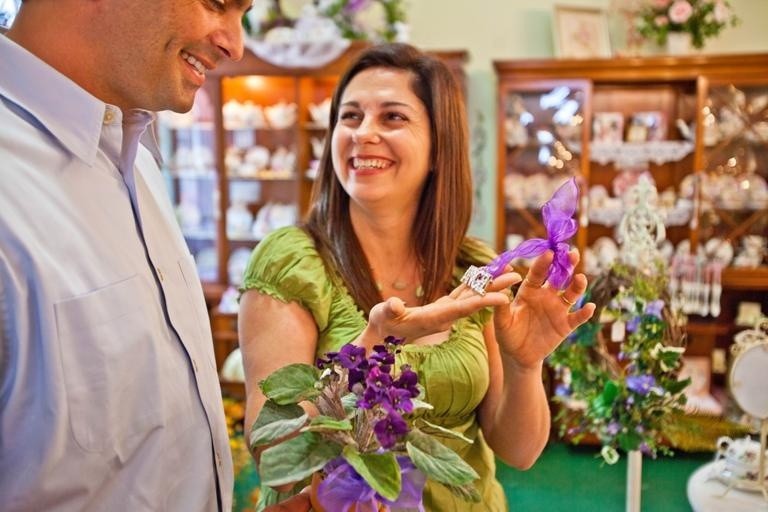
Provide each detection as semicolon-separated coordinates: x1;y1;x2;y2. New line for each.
629;1;742;50
482;171;581;292
543;253;695;469
248;334;484;512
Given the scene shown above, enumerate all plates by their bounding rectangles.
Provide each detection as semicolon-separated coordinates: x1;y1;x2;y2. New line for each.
498;97;768;270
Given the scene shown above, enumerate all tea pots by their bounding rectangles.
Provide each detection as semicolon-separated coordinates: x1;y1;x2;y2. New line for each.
714;435;767;482
162;98;331;290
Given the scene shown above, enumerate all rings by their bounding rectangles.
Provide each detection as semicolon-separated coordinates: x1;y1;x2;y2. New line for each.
561;295;575;307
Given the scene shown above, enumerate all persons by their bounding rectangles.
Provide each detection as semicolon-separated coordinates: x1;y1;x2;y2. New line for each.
235;40;597;511
1;0;254;510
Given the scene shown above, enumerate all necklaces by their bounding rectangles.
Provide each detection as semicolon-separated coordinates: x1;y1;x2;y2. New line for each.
367;250;425;299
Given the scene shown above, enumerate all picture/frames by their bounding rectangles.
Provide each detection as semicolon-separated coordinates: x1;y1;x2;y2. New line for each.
549;4;613;61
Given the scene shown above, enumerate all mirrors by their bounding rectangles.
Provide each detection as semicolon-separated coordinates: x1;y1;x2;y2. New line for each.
724;318;767;498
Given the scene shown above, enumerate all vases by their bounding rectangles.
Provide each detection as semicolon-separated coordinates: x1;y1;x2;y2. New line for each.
309;469;386;512
625;449;643;512
666;30;695;55
